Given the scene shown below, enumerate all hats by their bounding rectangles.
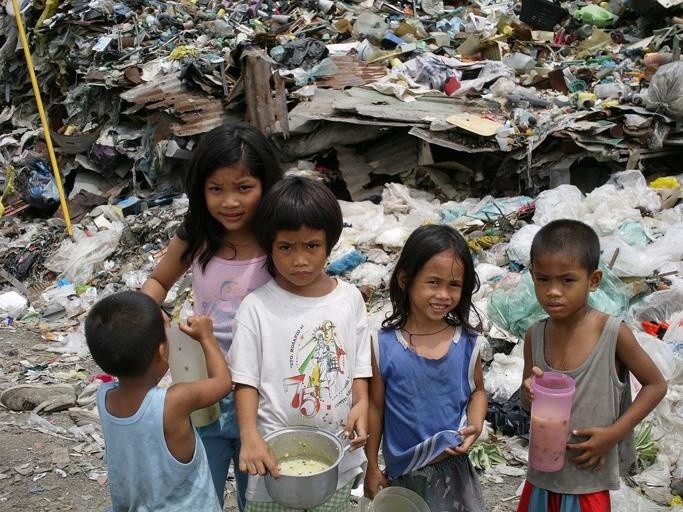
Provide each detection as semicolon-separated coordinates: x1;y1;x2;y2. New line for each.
422;0;443;15
611;31;630;44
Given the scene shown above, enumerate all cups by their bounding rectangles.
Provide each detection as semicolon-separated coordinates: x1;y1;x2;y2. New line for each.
529;371;576;472
368;484;432;512
166;322;222;427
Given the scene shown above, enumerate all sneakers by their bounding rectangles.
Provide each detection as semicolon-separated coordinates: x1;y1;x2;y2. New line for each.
69;408;102;432
0;384;76;411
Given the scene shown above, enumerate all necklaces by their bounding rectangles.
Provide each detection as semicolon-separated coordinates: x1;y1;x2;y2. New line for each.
548;310;589;370
402;325;451;348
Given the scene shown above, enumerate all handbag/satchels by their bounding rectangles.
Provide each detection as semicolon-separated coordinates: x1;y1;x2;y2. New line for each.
441;76;461;96
380;30;408;49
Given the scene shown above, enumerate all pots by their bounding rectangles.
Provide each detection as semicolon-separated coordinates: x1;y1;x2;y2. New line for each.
258;426;358;509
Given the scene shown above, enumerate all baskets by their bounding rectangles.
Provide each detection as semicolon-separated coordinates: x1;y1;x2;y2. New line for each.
519;0;569;31
45;96;106;154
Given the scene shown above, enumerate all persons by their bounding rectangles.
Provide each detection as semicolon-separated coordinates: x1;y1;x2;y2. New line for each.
517;220;667;512
225;174;374;512
141;122;285;512
363;224;488;512
85;290;231;512
